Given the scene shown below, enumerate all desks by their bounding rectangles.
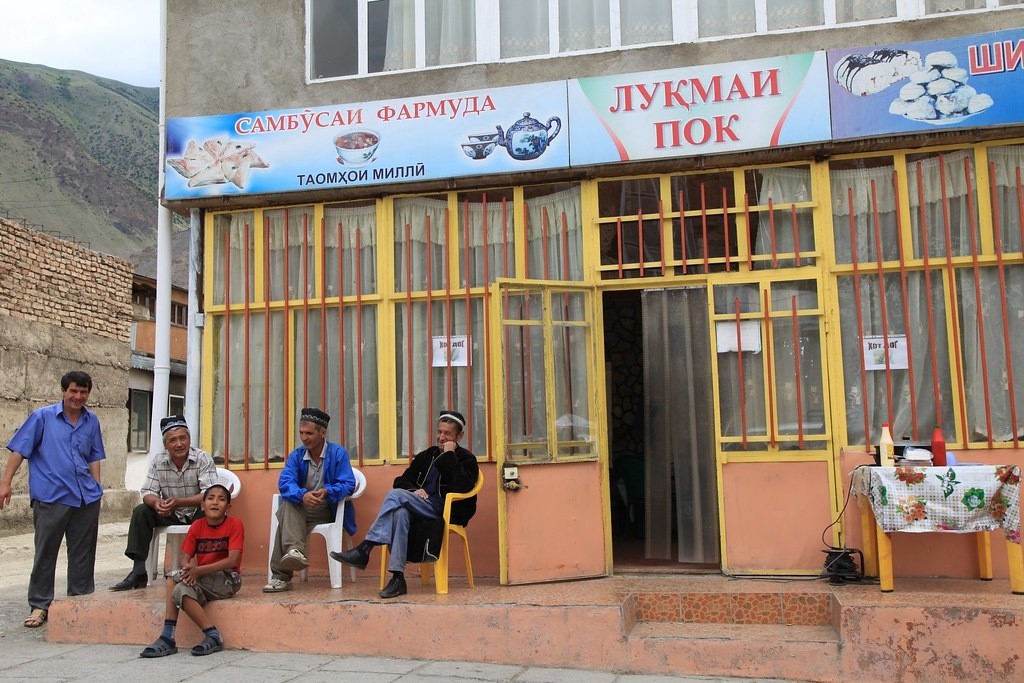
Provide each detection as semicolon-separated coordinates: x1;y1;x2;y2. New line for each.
848;464;1024;595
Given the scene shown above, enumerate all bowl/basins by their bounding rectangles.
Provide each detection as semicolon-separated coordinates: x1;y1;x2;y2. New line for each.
468;133;499;147
332;127;381;167
461;140;498;160
869;453;877;465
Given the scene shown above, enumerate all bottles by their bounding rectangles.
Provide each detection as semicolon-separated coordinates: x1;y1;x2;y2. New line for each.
931;424;946;466
880;423;894;467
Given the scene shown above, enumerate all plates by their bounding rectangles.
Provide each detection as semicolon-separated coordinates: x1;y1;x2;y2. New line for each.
903;108;987;126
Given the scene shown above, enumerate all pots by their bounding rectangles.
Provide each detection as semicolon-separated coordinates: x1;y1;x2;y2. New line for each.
876;435;931;466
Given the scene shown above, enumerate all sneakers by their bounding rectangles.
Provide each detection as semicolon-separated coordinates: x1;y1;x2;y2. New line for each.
263;579;293;593
279;548;310;571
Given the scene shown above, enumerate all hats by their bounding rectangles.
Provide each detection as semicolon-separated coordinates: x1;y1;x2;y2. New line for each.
438;410;466;429
160;415;188;435
300;408;330;429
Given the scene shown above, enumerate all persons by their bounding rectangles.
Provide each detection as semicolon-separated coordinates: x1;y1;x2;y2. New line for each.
109;415;218;592
0;371;107;628
140;484;245;658
263;409;356;592
329;410;478;598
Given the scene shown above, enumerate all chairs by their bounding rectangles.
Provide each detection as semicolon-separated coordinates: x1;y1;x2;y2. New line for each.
380;468;483;595
145;468;241;587
267;466;366;588
612;455;645;541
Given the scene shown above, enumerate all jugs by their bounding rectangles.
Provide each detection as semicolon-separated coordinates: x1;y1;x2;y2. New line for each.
496;112;561;160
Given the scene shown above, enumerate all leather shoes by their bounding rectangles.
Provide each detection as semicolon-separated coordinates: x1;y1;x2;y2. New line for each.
108;570;148;591
379;579;407;598
329;547;369;570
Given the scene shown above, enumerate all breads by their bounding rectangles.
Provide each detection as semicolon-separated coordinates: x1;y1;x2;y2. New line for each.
833;48;994;121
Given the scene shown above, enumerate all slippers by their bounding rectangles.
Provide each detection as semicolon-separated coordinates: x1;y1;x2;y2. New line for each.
24;608;47;627
191;637;223;655
140;639;178;657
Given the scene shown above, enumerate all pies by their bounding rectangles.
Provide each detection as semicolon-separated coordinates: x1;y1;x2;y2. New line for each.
166;135;269;190
